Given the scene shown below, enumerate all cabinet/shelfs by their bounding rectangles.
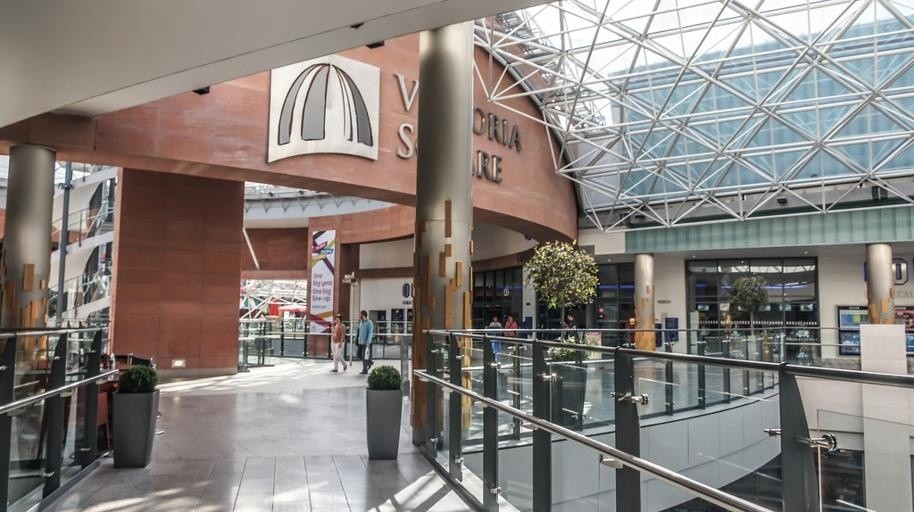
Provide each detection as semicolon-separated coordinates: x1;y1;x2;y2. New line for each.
836;305;914;357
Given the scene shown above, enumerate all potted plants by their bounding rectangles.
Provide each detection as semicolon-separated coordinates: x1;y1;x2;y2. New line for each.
545;331;601;430
365;364;404;460
109;364;160;469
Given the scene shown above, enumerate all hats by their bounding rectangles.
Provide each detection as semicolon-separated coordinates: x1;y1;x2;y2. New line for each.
335;314;341;317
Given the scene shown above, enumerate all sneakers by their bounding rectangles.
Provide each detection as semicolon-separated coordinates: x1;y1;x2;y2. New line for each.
368;361;373;369
329;369;337;372
360;370;368;374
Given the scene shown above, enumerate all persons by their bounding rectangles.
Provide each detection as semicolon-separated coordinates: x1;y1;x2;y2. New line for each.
488;315;519;361
563;314;579;336
330;310;375;374
